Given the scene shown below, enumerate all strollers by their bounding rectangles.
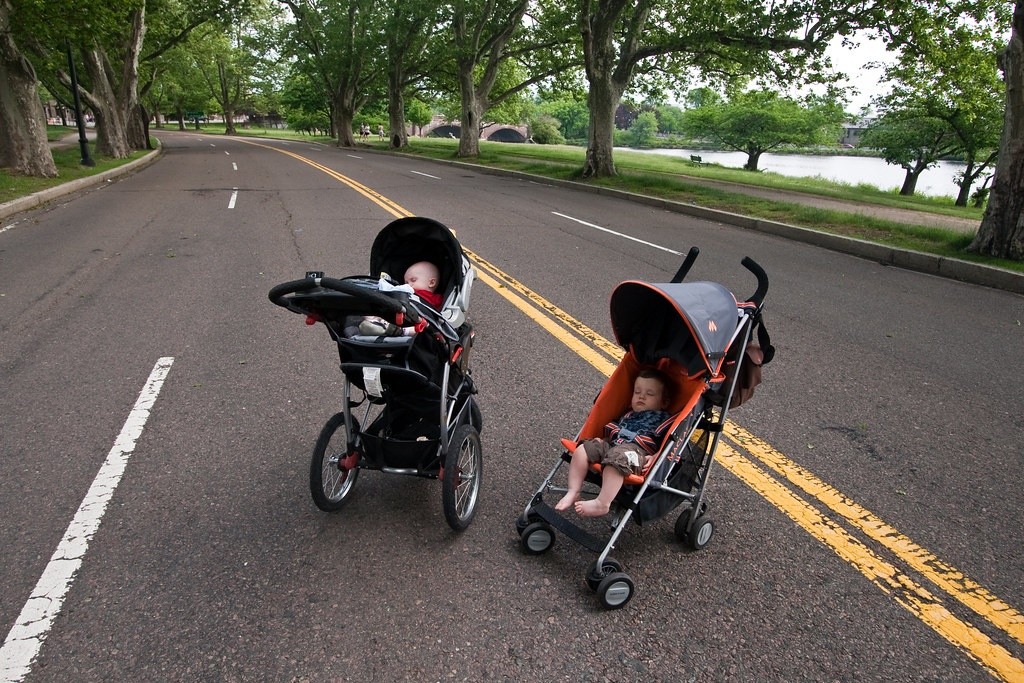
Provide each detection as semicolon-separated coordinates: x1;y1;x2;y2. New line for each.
268;217;483;534
516;247;775;610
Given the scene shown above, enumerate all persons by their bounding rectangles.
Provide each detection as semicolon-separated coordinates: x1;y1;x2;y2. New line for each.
344;261;442;337
554;368;676;520
